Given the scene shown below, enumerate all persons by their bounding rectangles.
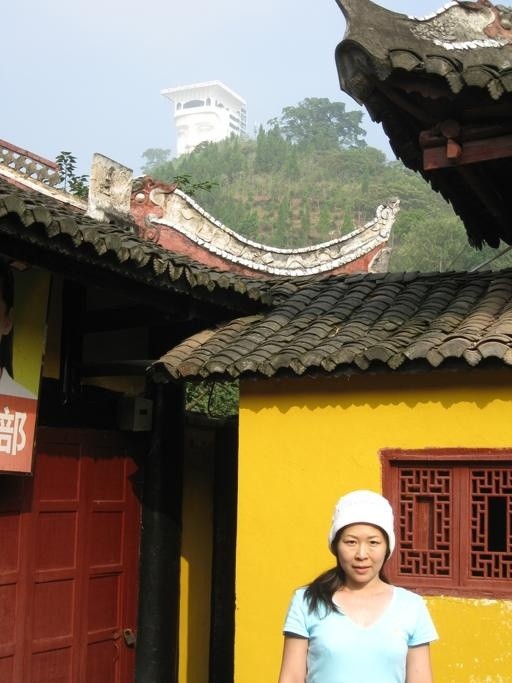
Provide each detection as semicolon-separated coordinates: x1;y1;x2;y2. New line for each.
277;491;440;683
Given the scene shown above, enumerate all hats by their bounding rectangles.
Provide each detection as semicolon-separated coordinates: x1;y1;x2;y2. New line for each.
329;490;396;561
